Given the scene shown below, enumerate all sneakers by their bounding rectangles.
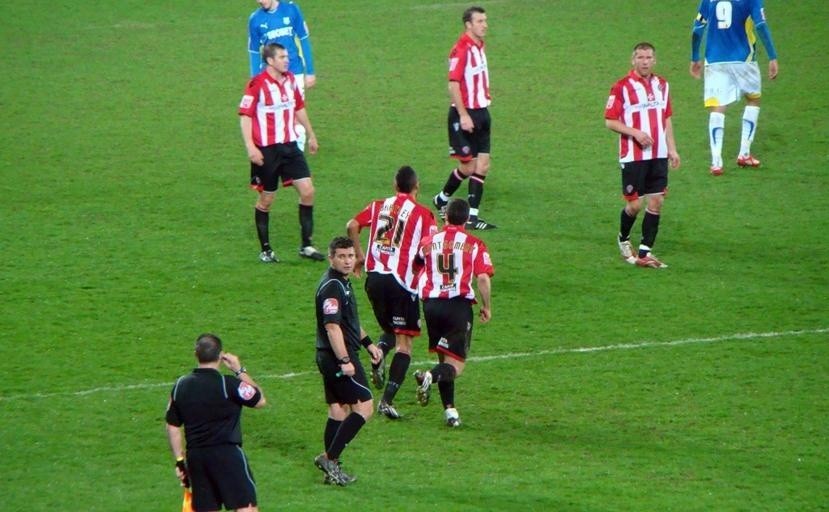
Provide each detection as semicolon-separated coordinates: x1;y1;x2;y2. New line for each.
316;455;346;486
636;251;667;269
618;236;638;263
443;406;462;427
299;245;326;260
738;156;761;168
261;247;280;263
711;166;725;176
371;355;384;390
414;369;431;406
324;471;356;485
434;194;448;223
465;219;497;229
377;401;401;419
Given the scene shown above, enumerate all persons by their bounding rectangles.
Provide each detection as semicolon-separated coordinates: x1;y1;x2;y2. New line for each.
169;334;264;512
606;43;682;271
316;235;385;484
432;7;503;232
416;197;499;428
349;166;441;420
691;0;778;177
238;44;326;263
245;1;315;92
